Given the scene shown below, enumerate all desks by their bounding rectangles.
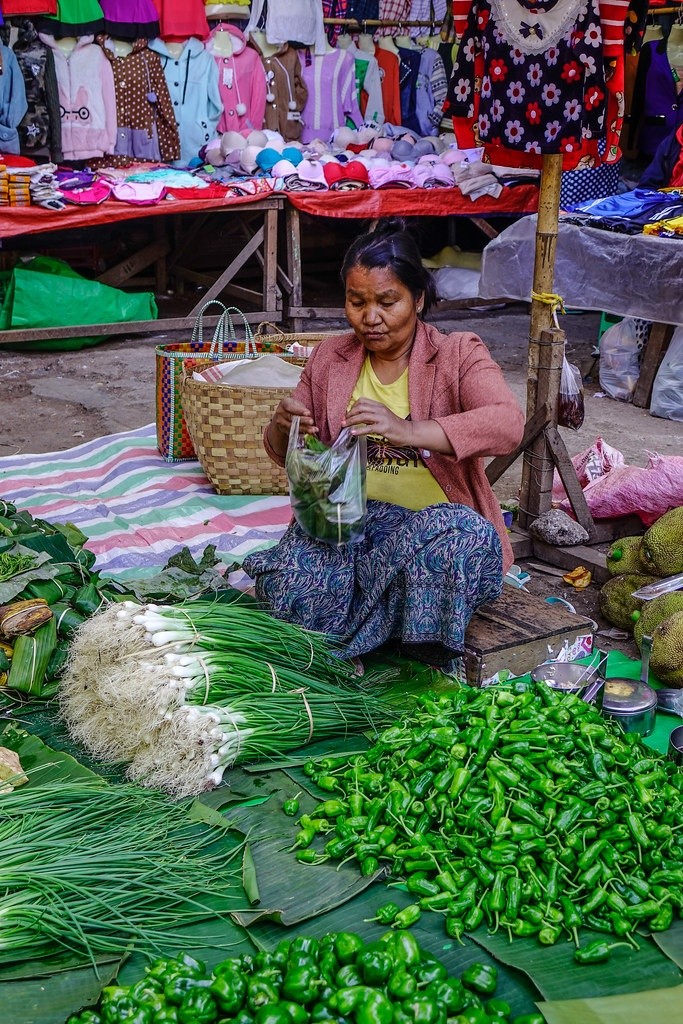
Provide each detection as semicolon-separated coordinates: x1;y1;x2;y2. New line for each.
480;203;683;414
283;184;539;333
0;194;291;347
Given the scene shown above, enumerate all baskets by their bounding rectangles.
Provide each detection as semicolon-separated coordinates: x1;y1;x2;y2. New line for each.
254;321;345;355
182;360;308;494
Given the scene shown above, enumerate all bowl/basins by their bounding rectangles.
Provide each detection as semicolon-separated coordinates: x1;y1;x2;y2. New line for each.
531;662;601;704
583;677;659;737
668;726;683;766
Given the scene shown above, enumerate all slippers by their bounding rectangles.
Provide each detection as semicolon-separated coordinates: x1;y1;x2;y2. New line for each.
502;565;531;593
545;597;598;632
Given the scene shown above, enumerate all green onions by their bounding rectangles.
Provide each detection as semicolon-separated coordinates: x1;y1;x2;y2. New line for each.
0;760;268;978
60;586;416;797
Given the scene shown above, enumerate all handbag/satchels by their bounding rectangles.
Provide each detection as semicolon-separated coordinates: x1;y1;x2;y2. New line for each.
153;299;295;462
285;413;368;547
559;353;586;434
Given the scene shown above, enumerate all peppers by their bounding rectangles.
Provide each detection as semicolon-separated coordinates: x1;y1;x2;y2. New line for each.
285;681;683;965
65;928;547;1024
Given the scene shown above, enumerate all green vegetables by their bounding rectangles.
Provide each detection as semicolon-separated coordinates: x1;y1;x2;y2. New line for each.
286;434;365;543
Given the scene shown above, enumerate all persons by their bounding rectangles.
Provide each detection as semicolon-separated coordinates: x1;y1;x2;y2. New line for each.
243;216;515;665
